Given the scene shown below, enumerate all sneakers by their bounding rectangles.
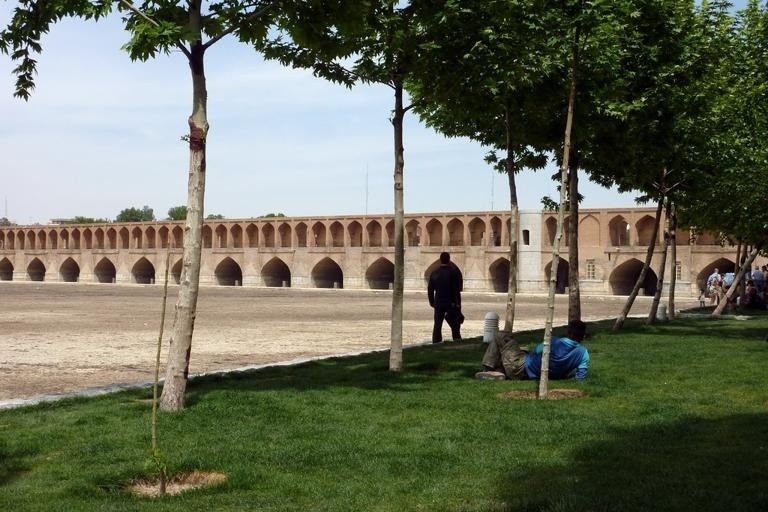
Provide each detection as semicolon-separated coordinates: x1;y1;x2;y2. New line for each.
475;371;505;380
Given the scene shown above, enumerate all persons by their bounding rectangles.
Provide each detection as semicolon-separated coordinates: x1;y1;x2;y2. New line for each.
476;319;592;386
697;264;768;311
426;251;466;343
